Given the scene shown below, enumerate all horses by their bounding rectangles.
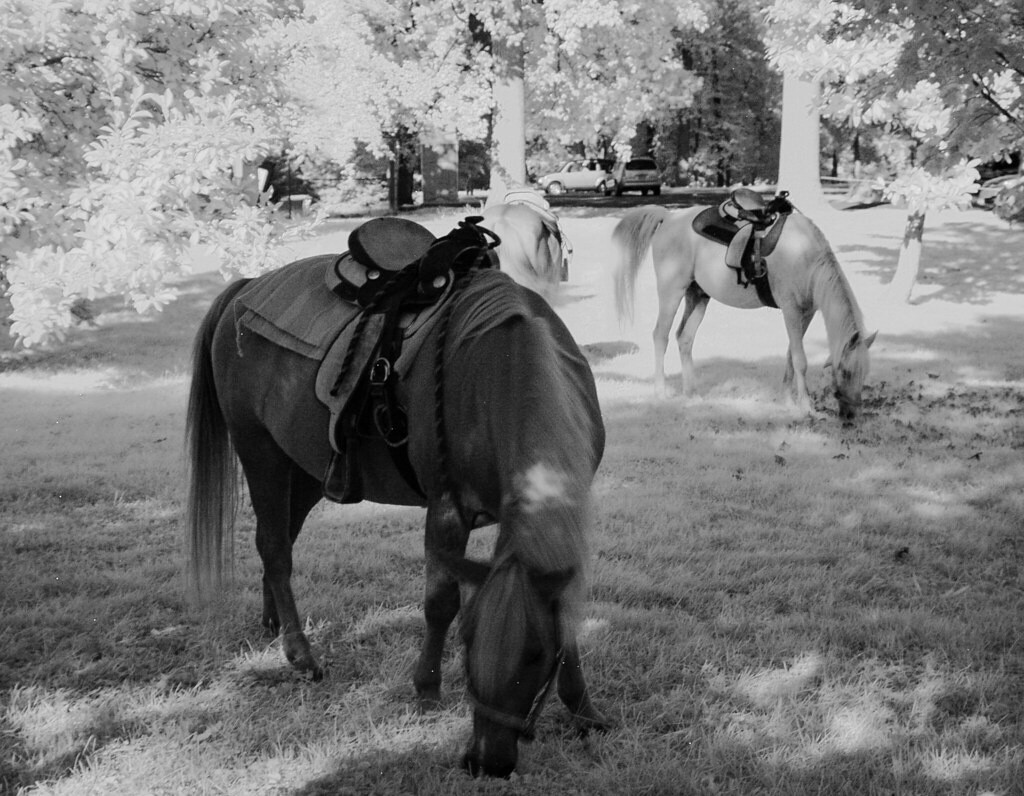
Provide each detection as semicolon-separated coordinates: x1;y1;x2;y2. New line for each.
182;253;610;780
479;204;564;307
609;203;877;426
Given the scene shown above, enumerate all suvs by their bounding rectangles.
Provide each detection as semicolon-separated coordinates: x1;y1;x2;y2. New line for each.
602;158;664;197
538;159;615;195
